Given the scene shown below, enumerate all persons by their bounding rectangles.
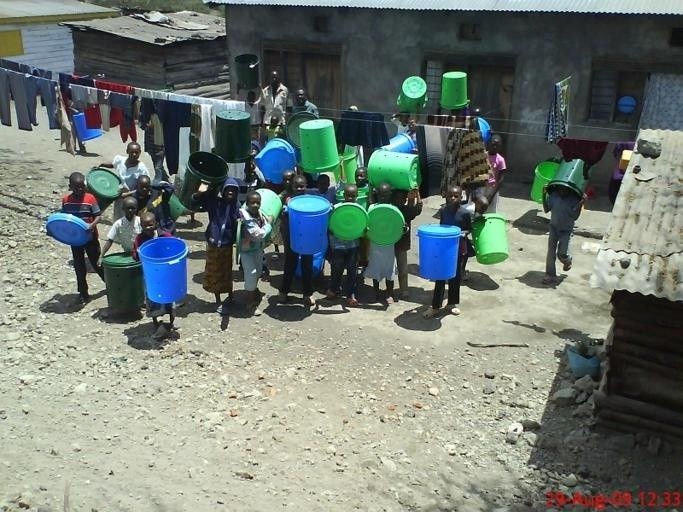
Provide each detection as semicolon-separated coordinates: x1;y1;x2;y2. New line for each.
423;101;505;317
203;152;423;315
45;142;199;338
253;71;318;147
391;102;421;153
542;184;589;285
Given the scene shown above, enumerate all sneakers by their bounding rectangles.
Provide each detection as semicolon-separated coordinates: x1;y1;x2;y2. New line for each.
423;307;440;317
563;259;572;270
543;275;555;284
326;290;335;295
446;303;459;315
460;271;469;280
345;296;359;305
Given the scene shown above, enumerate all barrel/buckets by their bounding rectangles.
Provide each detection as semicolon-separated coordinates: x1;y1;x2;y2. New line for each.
72;53;589;311
